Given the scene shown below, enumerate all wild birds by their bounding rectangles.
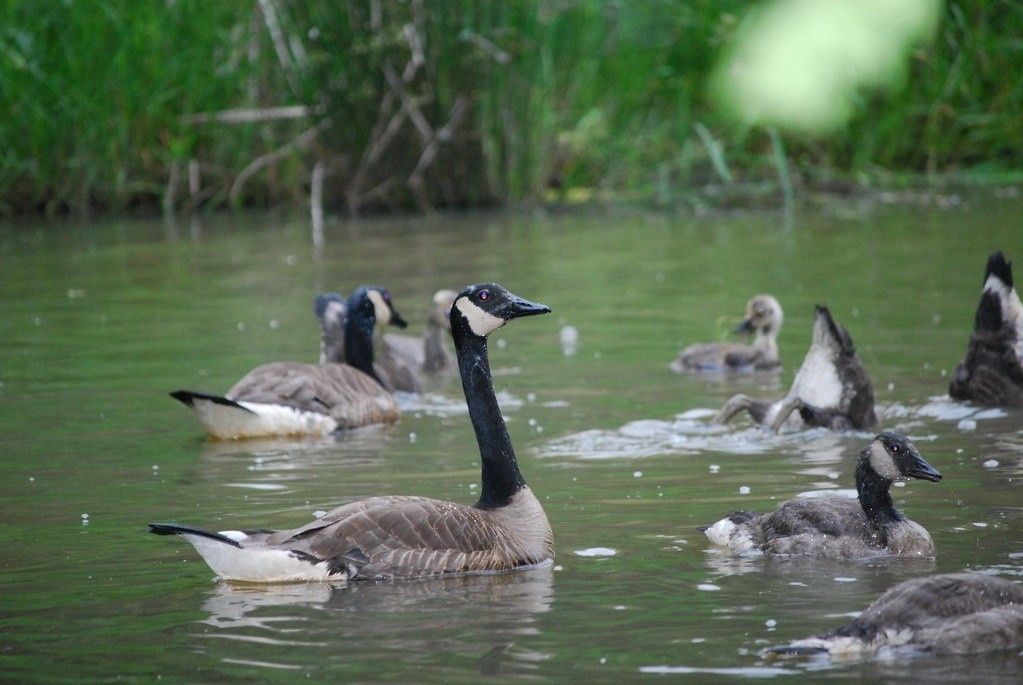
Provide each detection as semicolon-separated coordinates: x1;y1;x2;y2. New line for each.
146;252;1023;660
